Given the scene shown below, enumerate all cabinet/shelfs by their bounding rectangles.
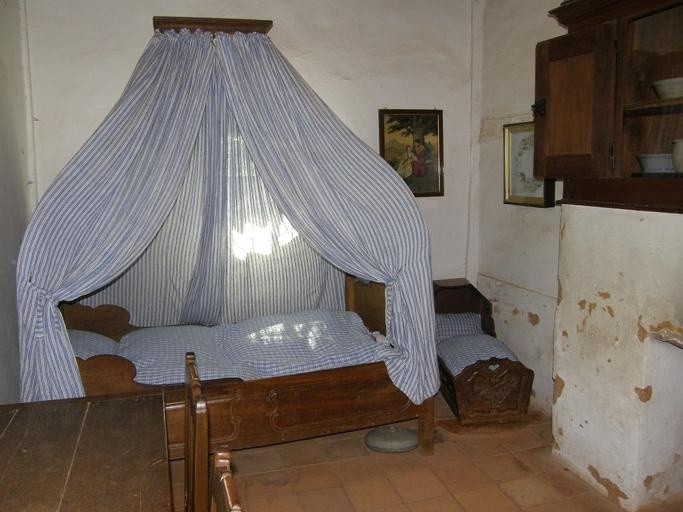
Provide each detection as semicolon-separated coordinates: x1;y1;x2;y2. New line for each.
533;1;683;214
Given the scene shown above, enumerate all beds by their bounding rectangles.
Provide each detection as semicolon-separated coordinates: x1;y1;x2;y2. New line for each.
57;274;434;462
433;278;535;426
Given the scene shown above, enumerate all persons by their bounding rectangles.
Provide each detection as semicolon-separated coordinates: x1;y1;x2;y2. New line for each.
394;144;418;180
411;138;425;178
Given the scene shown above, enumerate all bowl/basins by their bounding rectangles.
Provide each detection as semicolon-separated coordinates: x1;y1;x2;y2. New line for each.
635;155;675;173
652;77;682;101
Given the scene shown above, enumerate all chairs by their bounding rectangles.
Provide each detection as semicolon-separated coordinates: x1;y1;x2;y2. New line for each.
170;353;210;512
210;447;243;512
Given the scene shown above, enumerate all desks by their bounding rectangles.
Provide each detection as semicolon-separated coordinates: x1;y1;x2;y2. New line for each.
0;392;172;512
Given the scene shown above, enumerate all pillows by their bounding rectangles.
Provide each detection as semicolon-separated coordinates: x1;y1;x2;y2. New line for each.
436;312;483;344
67;327;118;360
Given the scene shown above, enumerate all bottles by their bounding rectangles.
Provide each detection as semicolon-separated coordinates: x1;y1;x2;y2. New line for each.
672;138;683;173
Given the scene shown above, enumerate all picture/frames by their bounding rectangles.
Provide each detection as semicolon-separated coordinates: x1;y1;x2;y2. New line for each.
503;122;555;208
378;107;445;197
